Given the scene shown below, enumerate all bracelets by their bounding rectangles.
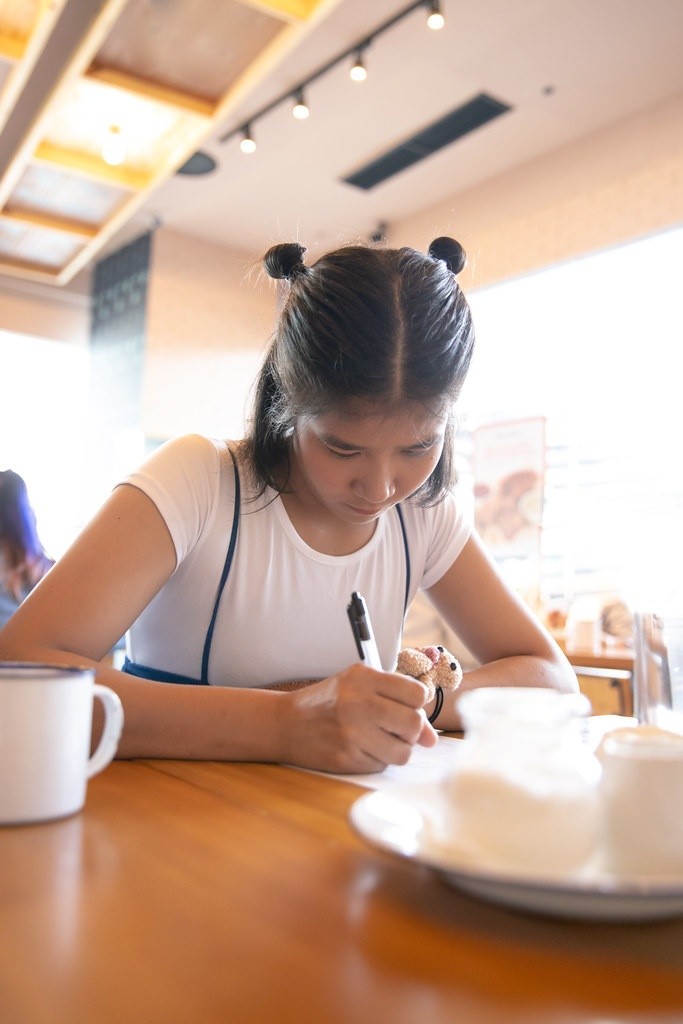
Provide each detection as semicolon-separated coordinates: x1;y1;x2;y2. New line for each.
397;643;463;724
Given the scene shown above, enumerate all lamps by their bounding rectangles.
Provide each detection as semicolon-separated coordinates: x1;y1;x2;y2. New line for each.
424;0;444;30
350;47;367;81
240;124;257;154
292;87;309;119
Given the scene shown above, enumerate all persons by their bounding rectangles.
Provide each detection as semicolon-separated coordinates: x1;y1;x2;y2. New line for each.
0;469;114;669
0;236;583;776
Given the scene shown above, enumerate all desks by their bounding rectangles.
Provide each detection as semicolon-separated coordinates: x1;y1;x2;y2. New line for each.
0;731;683;1024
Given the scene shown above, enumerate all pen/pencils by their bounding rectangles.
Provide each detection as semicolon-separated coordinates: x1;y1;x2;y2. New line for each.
347;590;383;674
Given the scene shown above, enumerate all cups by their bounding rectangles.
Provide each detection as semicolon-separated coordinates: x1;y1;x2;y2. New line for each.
0;660;124;825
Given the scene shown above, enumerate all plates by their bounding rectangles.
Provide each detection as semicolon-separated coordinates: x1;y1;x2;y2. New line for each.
348;789;683;923
